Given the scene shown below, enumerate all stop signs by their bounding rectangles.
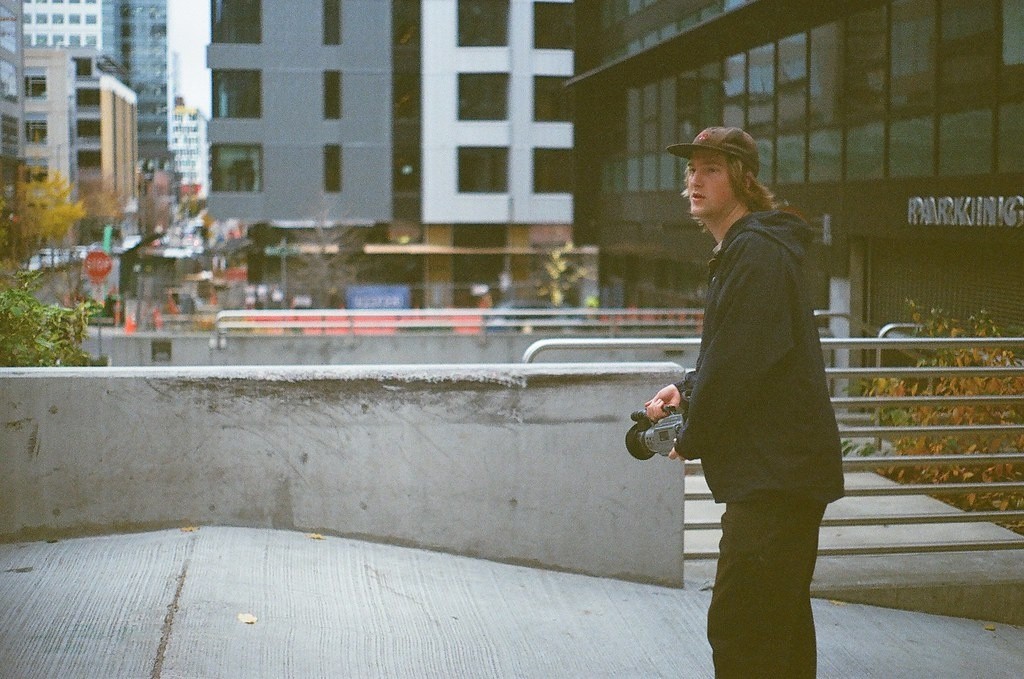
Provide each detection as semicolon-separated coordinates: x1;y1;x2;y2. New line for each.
85;251;112;281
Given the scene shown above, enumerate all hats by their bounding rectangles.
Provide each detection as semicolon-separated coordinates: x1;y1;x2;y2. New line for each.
665;126;759;178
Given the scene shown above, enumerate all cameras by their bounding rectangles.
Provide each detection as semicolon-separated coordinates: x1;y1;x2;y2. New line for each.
625;388;693;460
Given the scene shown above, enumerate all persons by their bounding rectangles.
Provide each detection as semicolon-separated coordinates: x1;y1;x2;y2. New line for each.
641;123;848;679
